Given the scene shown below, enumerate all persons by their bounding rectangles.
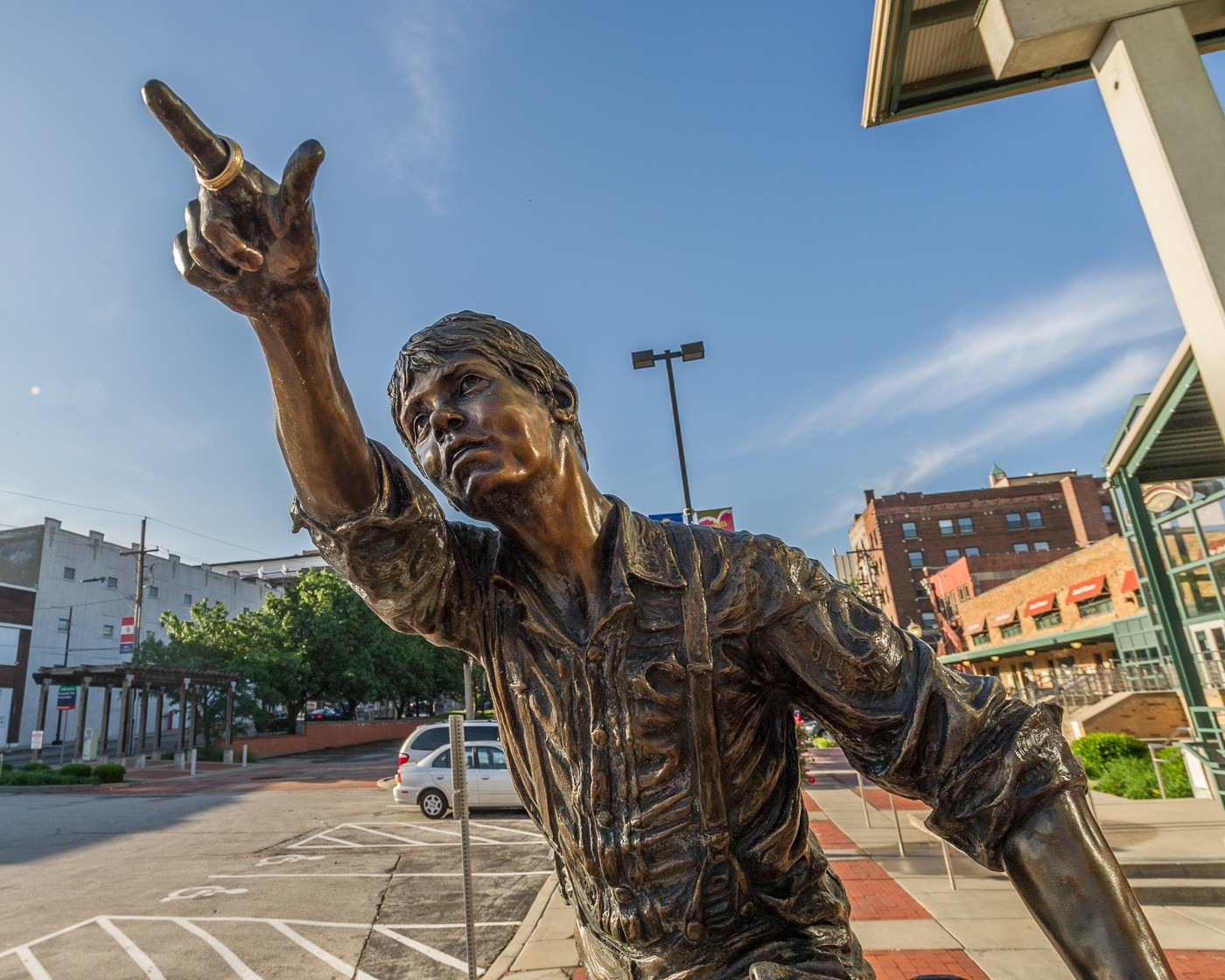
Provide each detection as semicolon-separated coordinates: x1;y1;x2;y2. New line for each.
140;78;1176;980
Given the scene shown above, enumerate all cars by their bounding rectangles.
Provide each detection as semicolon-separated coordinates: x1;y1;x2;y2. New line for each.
305;708;341;720
267;711;288;729
393;740;528;820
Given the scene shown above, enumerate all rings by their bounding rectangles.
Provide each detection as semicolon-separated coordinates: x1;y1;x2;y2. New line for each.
195;133;244;191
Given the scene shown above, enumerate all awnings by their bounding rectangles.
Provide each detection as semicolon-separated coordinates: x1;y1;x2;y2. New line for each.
1122;559;1166;593
1066;575;1110;603
1200;538;1225;566
1024;593;1059;617
968;618;987;635
992;607;1020;626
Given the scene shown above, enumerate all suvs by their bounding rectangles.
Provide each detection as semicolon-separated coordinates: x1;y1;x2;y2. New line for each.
398;719;502;767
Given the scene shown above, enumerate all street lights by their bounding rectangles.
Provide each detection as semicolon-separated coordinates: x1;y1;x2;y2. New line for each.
631;340;704;524
81;576;141;755
